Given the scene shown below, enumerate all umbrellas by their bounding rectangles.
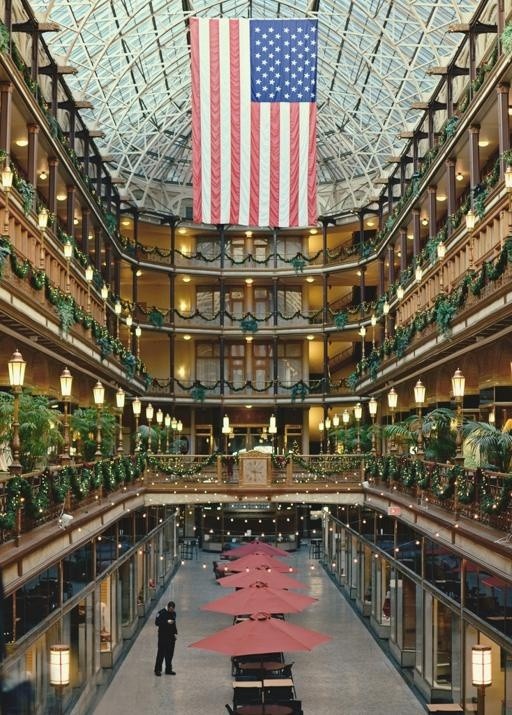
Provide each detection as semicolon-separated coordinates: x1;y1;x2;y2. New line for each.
188;538;333;711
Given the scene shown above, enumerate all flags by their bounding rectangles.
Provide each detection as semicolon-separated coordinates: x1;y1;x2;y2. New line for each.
190;16;320;228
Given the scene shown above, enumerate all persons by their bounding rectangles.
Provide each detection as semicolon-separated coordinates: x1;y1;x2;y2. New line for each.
155;601;177;676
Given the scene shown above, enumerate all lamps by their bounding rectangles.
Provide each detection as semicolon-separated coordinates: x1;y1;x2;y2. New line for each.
74;212;144;276
2;166;73;262
471;645;492;710
414;380;426;403
85;265;93;282
60;367;72;403
452;369;464;399
438;241;445;260
387;388;398;408
357;300;389;338
466;210;475;230
456;173;463;181
102;285;142;338
415;265;422;283
436;194;447;201
93;380;104;408
397;286;403;301
8;347;26;388
505;168;512;191
116;387;125;409
355;220;429;276
133;396;183;434
45;643;72;715
318;396;378;434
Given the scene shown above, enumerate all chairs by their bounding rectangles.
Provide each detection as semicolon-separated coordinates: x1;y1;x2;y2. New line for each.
225;614;303;715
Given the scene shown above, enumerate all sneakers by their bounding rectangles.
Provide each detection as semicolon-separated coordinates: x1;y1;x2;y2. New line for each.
155;669;161;676
165;670;177;675
